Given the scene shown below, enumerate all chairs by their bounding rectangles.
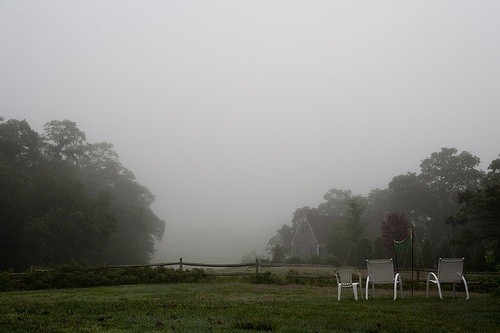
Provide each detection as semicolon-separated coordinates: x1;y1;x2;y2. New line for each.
365;258;404;300
334;265;364;301
426;257;470;301
